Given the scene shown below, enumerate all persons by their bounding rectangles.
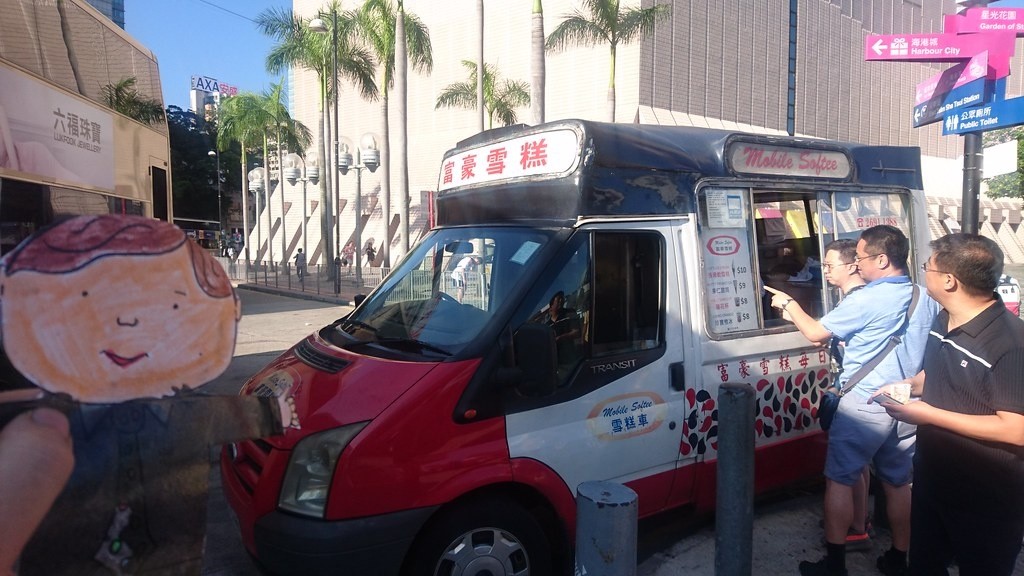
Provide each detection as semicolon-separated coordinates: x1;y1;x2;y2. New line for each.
451;256;483;304
218;228;229;256
233;231;242;257
293;248;306;282
366;242;376;266
867;234;1024;576
544;290;582;345
344;241;355;274
763;230;945;576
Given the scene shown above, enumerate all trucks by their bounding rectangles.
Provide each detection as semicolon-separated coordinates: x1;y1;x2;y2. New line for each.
217;115;932;575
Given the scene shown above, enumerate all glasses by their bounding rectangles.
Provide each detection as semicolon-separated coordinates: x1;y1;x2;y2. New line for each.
921;261;965;285
822;262;852;269
854;252;886;262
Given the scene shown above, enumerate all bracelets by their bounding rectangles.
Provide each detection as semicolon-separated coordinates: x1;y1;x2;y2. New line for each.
781;298;794;309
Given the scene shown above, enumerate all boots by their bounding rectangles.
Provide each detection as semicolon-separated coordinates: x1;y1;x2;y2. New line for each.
798;542;849;576
876;545;908;576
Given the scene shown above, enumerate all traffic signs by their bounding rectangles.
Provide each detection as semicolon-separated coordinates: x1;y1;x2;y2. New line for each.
914;48;1009;104
910;77;994;131
940;0;1024;36
943;95;1024;135
865;34;1019;57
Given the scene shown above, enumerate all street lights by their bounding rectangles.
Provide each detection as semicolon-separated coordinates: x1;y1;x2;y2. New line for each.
338;136;381;291
247;167;267;270
283;152;320;276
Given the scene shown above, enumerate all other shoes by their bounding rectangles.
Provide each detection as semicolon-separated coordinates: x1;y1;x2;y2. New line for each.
820;529;873;552
865;523;876;539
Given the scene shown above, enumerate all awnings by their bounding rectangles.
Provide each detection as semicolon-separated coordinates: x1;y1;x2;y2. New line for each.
778;208;847;241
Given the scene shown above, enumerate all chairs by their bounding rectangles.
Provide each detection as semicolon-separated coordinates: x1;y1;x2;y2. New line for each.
624;256;658;334
